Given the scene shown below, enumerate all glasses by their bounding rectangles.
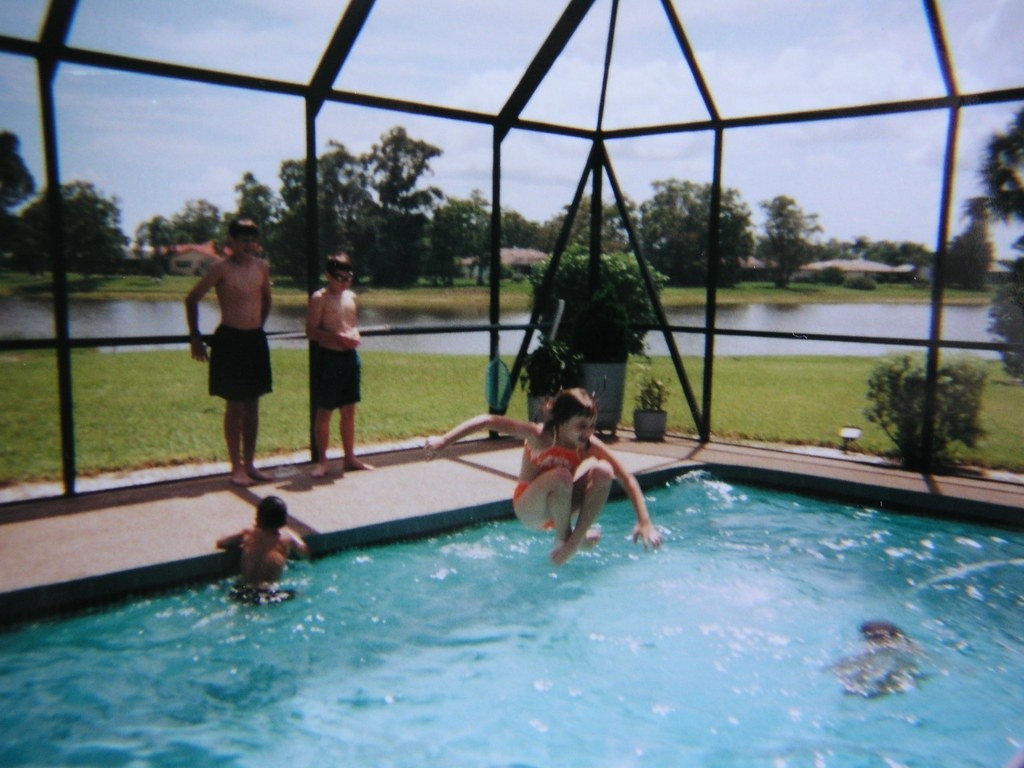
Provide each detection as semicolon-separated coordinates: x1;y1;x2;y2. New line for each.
331;273;353;283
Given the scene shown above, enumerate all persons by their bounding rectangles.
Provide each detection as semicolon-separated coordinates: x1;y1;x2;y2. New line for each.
424;387;668;567
216;495;308;604
304;252;378;479
183;219;274;487
820;622;961;698
